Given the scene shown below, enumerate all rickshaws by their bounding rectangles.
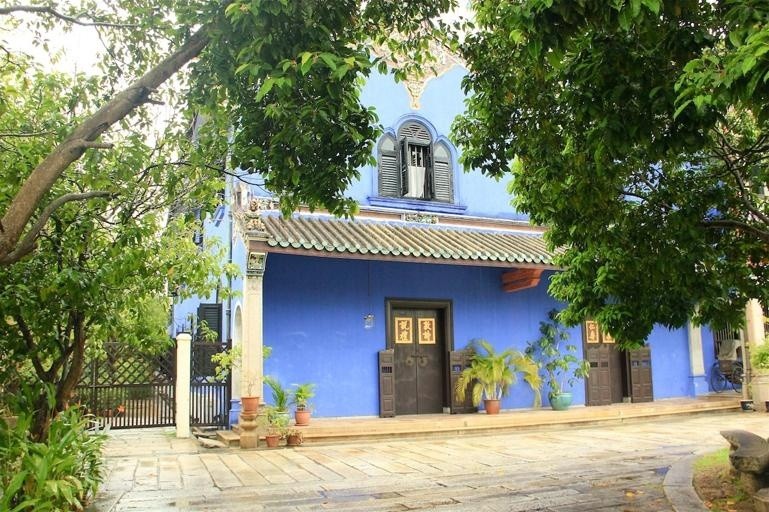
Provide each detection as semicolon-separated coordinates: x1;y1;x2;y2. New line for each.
711;359;744;393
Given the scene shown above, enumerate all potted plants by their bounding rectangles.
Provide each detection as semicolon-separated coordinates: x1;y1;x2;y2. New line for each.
240;371;260;414
293;384;316;425
263;375;291;425
749;335;769;402
455;337;545;414
525;308;589;409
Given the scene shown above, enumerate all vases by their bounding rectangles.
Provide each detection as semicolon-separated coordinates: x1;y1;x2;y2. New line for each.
287;435;298;445
266;435;281;447
